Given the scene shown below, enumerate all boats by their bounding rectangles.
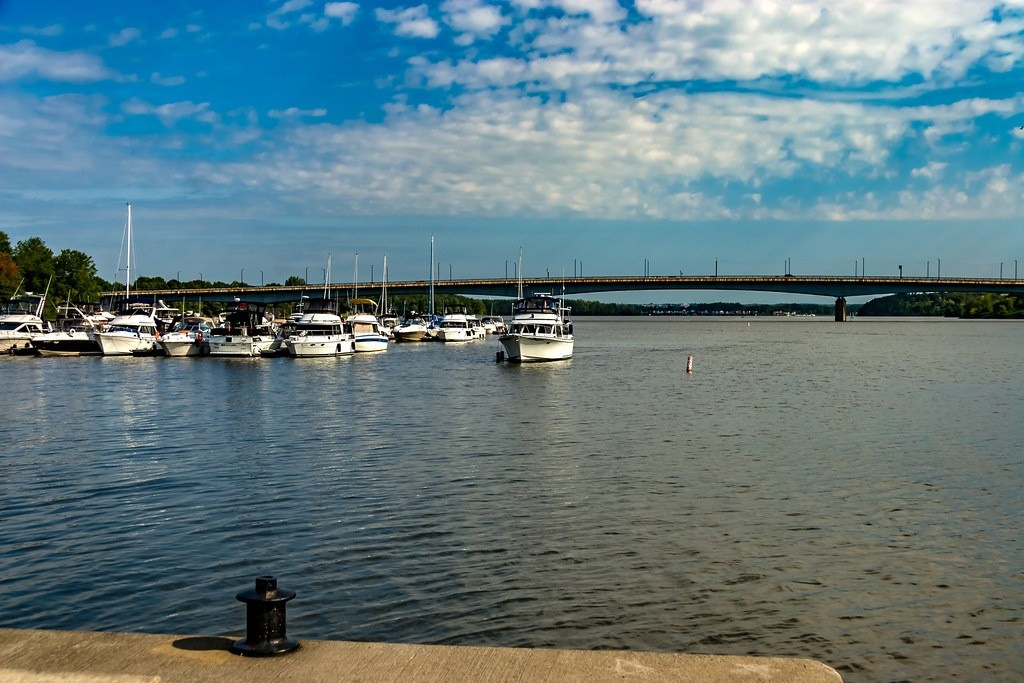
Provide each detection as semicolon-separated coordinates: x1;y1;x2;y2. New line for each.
0;202;511;360
496;246;574;362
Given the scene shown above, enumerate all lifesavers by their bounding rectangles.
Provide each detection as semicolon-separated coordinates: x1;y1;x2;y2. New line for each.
197;331;203;342
69;328;76;337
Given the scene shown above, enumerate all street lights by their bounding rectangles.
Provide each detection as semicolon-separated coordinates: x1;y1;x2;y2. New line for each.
928;260;929;277
863;257;864;278
514;262;516;279
1000;261;1003;278
936;258;944;279
788;256;791;274
575;258;577;278
581;260;583;278
546;266;550;279
784;259;787;275
645;257;646;277
1013;259;1018;280
715;257;718;277
855;259;859;277
647;259;650;276
